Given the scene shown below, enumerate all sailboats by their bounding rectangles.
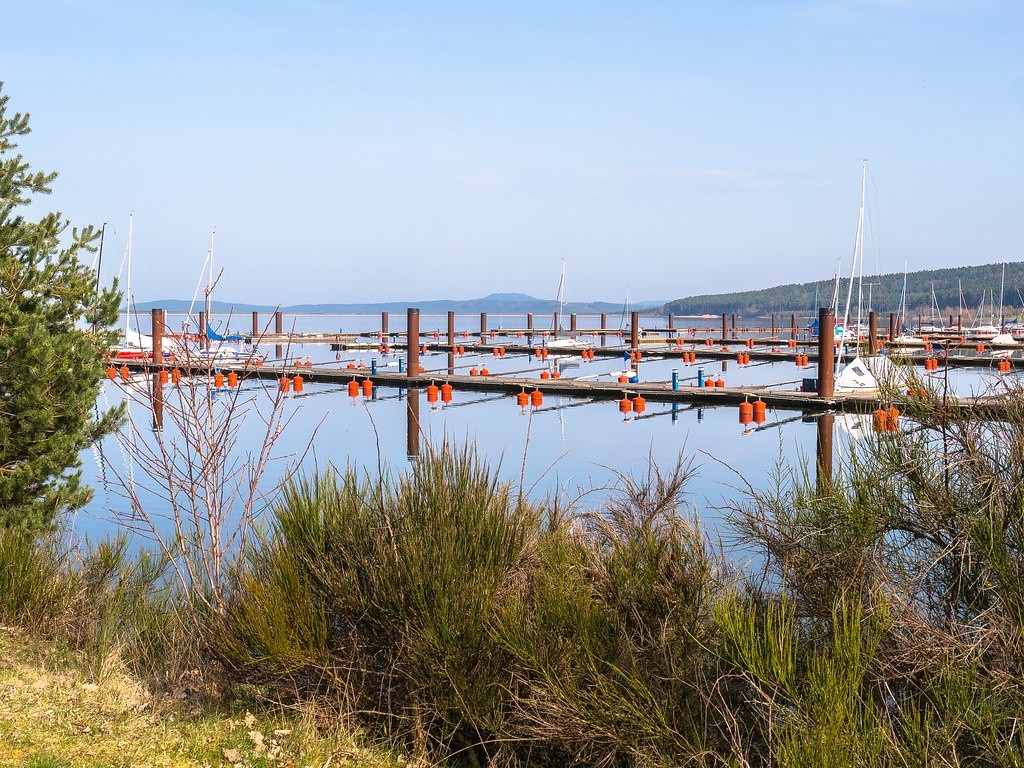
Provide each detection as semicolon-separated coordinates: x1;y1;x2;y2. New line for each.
521;256;593;349
93;212;266;368
807;155;1023;392
616;287;642;337
